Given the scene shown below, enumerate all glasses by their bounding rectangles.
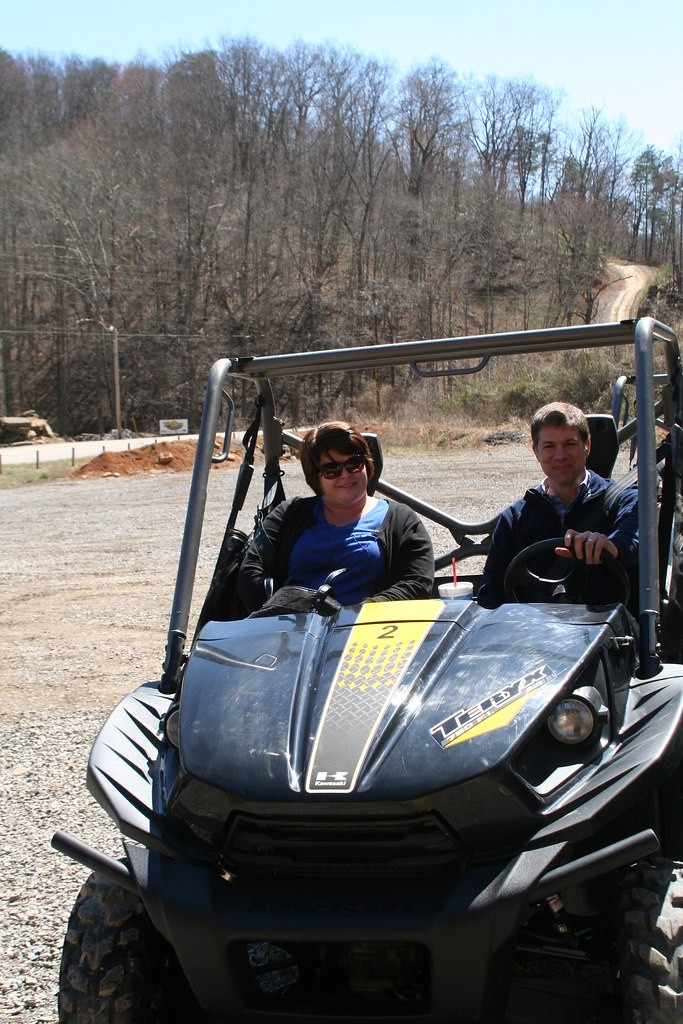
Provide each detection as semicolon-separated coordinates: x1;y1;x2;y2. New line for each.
315;455;365;479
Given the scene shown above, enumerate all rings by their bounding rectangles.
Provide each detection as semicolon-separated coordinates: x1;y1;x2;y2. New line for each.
588;538;595;544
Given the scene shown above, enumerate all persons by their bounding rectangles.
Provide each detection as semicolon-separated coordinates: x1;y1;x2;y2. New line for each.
238;421;435;615
477;402;639;610
